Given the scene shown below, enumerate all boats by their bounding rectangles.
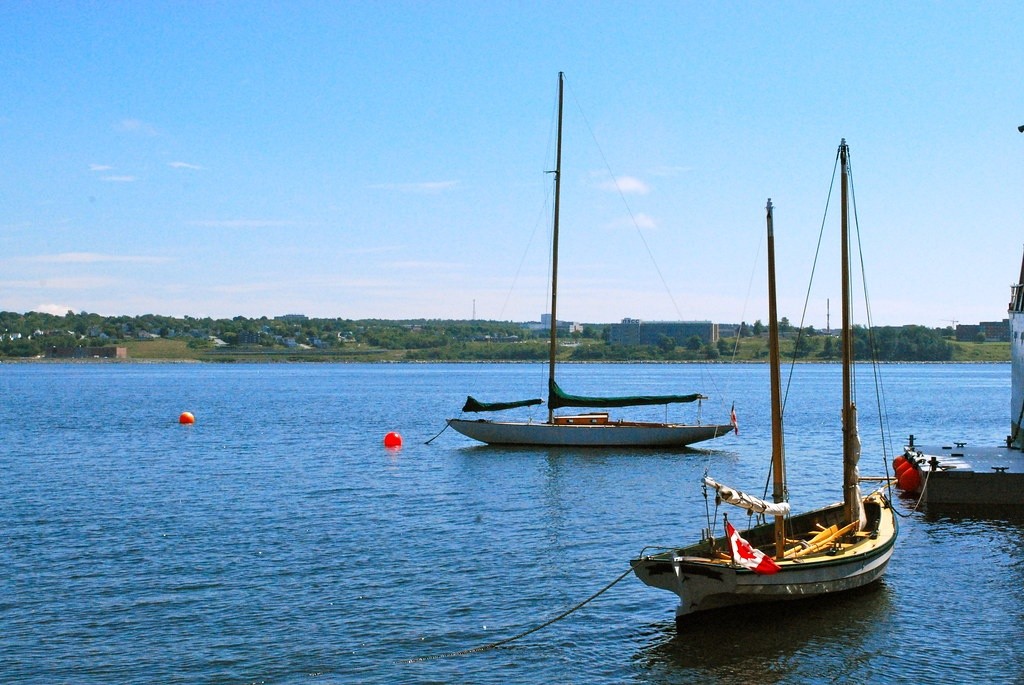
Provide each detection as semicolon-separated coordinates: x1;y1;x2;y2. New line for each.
893;123;1024;513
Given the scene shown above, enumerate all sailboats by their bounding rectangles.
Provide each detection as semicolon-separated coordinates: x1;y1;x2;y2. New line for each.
629;138;901;623
445;71;736;446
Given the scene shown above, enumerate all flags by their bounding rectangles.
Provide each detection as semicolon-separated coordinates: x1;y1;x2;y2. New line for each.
727;522;781;575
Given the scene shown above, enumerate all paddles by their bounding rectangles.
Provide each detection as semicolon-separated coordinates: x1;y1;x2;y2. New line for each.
794;520;860;557
768;525;838;560
